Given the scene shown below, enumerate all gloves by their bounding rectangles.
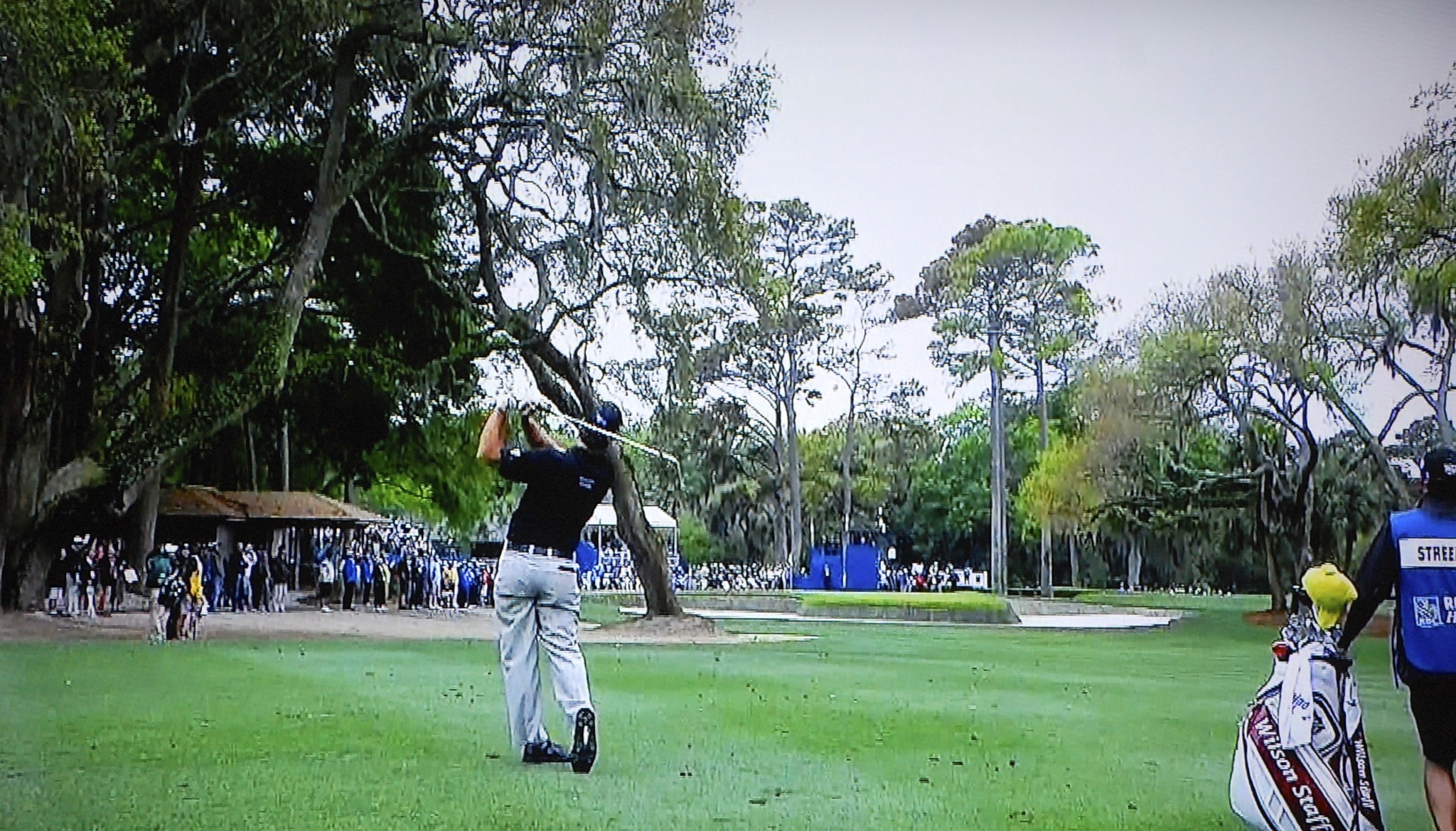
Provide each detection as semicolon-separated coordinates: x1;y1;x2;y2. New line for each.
518;398;545;417
493;393;518;421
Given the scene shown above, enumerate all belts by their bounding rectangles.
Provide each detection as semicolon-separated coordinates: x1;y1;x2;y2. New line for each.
506;541;572;559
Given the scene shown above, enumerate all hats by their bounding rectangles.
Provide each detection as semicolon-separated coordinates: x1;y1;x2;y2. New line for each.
1420;447;1456;492
580;401;622;442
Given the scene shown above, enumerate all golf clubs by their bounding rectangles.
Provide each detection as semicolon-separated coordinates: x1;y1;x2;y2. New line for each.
1269;612;1348;663
506;395;689;493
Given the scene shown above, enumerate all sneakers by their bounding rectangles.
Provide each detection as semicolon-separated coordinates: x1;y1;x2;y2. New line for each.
568;706;598;774
520;737;571;765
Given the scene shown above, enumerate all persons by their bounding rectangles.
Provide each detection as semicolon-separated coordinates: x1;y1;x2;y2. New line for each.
306;515;497;613
43;532;292;647
577;538;792;594
874;558;960;595
476;387;624;774
963;567;971;586
1336;447;1456;831
